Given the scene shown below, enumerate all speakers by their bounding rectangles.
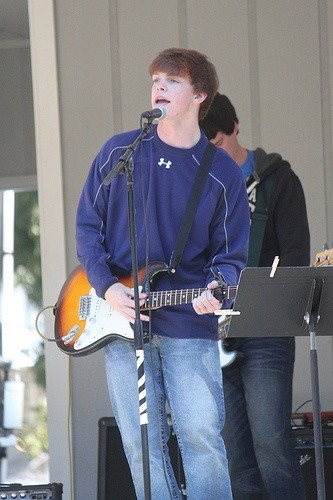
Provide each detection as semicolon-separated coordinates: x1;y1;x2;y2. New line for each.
290;430;333;500
96;417;188;500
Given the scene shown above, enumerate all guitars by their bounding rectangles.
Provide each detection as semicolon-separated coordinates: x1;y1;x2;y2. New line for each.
54;264;243;357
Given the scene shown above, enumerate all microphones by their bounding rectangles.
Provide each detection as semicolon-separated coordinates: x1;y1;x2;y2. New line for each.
141;106;167;120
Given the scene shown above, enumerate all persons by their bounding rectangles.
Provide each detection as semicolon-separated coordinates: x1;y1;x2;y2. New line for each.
75;49;250;500
198;91;310;500
